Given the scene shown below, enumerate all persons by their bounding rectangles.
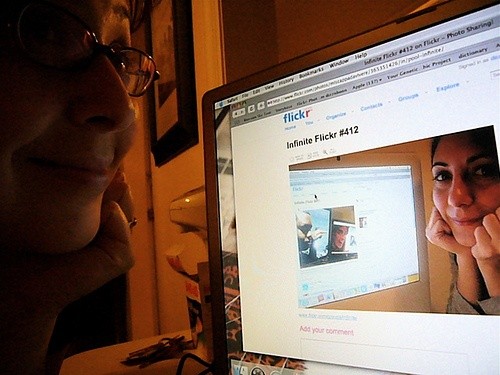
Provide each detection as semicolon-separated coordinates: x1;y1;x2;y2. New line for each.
424;125;500;315
296;211;322;266
330;224;349;252
0;0;162;360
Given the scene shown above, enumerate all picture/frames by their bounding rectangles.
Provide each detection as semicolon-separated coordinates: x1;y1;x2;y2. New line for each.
141;0;197;166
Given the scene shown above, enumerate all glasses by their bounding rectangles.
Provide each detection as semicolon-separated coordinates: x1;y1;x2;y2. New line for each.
14;2;161;96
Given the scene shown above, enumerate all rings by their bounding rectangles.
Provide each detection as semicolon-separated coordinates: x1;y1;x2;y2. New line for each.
128;216;137;229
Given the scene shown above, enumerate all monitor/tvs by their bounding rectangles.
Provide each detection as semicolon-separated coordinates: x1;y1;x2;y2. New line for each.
287;152;431;314
202;0;500;375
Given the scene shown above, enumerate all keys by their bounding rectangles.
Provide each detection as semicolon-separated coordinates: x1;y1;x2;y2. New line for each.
119;332;198;369
